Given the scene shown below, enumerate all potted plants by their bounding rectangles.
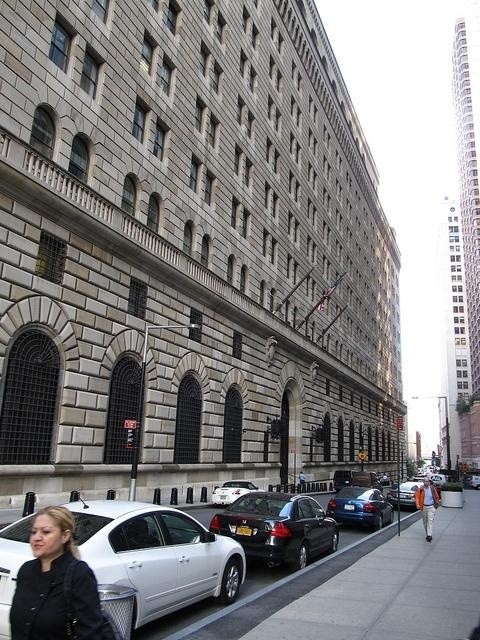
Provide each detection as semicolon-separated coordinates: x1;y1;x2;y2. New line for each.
441;481;465;508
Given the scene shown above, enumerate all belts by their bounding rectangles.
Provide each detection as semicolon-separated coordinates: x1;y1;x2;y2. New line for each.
424;504;434;506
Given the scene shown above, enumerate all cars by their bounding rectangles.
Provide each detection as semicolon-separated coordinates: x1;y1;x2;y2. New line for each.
0;499;245;640
326;485;395;531
209;491;340;572
212;480;267;508
394;475;446;510
464;469;480;490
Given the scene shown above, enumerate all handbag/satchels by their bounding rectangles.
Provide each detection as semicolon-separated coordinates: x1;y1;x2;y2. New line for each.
62;559;123;640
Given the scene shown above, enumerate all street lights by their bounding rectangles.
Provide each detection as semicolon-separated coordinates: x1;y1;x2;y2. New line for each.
412;396;450;475
129;324;200;501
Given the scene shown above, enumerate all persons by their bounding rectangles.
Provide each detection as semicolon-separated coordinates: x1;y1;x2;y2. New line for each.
299;471;305;484
414;477;440;542
10;506;103;640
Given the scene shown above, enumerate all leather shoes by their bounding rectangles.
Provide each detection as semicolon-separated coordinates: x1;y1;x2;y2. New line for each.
425;536;432;542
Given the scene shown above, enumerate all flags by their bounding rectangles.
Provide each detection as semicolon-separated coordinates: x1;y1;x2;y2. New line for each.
318;284;337;310
